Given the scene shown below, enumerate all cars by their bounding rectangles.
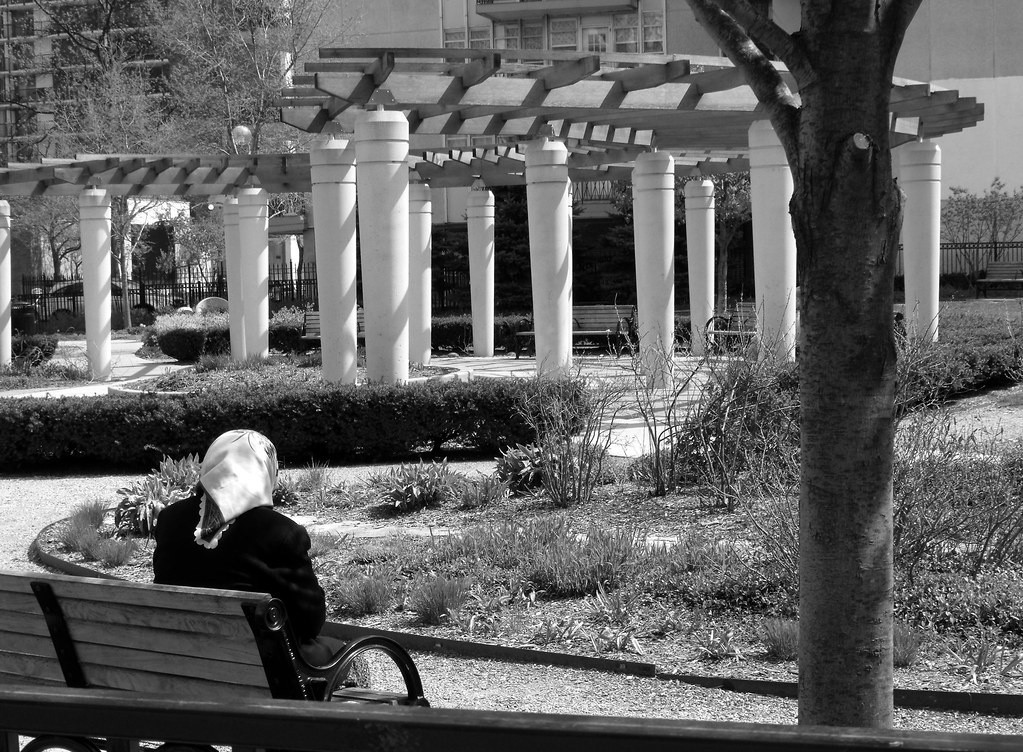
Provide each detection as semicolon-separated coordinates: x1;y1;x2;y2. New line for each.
12;301;41;338
32;280;183;331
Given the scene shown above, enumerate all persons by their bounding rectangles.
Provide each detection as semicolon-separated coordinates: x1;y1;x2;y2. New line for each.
154;429;350;695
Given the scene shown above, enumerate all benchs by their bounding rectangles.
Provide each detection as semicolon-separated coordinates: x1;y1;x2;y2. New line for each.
0;570;430;710
299;307;366;353
706;302;757;350
514;305;636;358
976;263;1023;297
